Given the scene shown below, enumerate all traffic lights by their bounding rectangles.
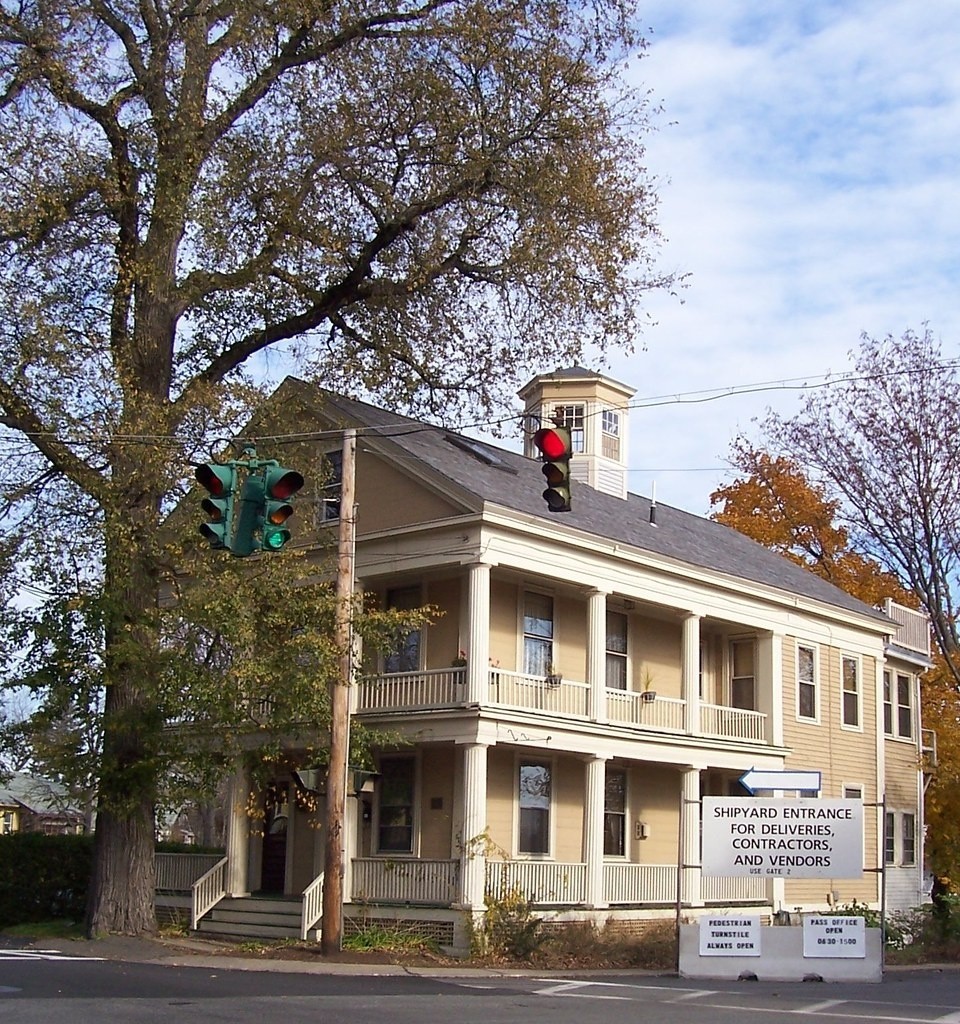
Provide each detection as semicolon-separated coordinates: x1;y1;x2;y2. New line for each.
256;463;305;553
195;462;237;551
532;422;576;514
